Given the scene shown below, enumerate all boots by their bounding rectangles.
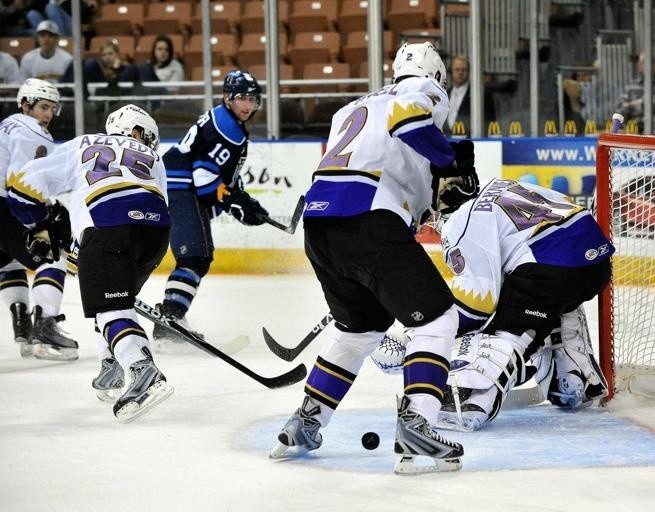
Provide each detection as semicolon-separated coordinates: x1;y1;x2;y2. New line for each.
438;358;517;427
545;309;611;408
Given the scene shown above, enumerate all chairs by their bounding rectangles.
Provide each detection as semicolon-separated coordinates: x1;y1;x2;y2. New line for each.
0;0;469;139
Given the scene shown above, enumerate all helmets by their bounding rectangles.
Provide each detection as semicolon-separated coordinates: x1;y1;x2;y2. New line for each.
15;77;62;110
390;40;450;87
105;103;159;143
222;69;261;97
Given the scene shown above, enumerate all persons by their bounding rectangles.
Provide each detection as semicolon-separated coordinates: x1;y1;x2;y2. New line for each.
0;77;79;349
11;103;172;416
0;0;185;116
153;70;269;341
278;42;655;455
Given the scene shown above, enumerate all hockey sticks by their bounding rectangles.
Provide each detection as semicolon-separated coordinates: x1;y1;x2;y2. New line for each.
255;195;305;235
262;311;334;361
71;242;249;357
58;248;307;388
502;112;594;411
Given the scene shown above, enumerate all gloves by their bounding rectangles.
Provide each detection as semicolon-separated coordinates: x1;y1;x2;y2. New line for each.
428;137;483;216
216;186;271;227
24;197;75;265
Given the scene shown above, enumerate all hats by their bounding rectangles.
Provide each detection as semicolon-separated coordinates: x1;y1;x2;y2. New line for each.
35;20;62;35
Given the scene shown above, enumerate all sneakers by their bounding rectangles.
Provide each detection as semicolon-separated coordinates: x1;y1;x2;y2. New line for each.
392;393;466;459
31;304;80;349
111;345;167;417
149;298;207;344
91;346;126;391
277;394;325;451
8;301;34;344
